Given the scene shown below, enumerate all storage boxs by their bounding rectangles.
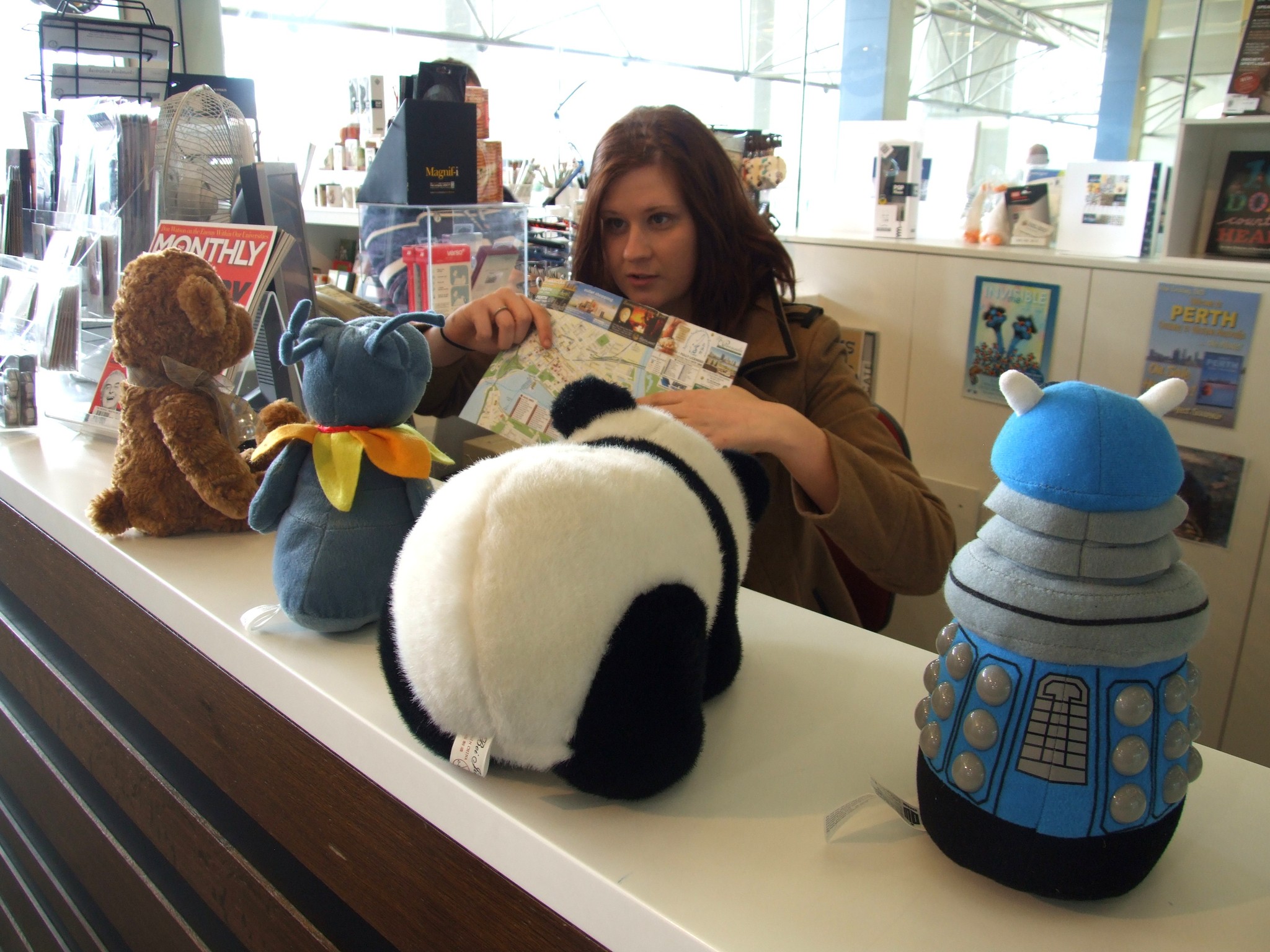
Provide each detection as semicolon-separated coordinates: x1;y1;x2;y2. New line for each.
357;201;528;317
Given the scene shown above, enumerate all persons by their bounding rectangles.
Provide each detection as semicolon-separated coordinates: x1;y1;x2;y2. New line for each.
100;370;126;412
432;57;516;202
419;105;956;630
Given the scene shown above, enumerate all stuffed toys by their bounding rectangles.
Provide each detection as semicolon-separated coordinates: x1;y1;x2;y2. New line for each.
379;373;773;802
248;299;457;636
916;370;1211;905
90;247;307;536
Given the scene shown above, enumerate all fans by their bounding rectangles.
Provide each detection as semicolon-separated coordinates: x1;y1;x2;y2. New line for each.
155;85;255;223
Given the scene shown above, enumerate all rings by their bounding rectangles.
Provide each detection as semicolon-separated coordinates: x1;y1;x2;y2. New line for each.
492;306;507;320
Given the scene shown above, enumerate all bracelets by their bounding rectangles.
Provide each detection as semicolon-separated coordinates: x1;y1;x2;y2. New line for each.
440;328;476;351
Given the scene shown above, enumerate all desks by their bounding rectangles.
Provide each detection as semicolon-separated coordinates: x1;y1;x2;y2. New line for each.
0;423;1270;952
